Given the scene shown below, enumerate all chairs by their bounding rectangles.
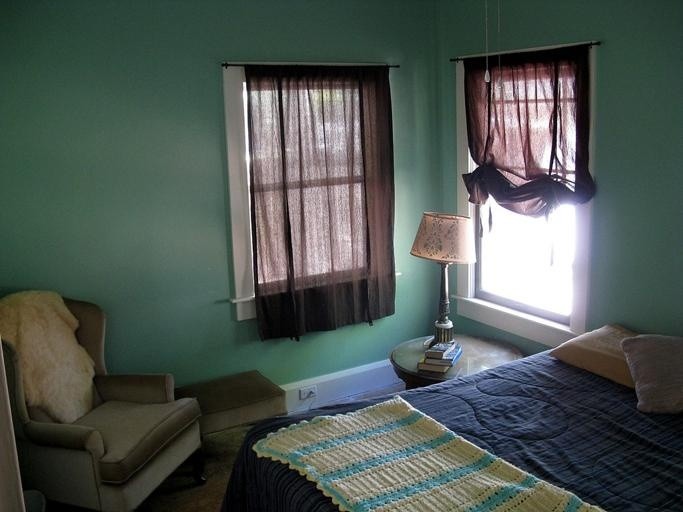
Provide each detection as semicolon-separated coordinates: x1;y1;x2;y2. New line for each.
0;284;208;512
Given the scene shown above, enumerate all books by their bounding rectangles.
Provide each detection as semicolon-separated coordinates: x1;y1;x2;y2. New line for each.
417;341;464;373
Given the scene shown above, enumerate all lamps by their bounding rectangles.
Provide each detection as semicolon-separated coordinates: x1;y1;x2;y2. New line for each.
407;206;476;353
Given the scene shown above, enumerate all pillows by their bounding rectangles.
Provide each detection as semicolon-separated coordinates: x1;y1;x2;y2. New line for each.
550;323;683;426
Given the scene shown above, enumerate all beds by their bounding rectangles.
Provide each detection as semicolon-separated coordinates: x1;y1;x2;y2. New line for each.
234;321;683;511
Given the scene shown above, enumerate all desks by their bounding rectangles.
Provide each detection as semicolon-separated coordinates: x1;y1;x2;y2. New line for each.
388;334;530;394
